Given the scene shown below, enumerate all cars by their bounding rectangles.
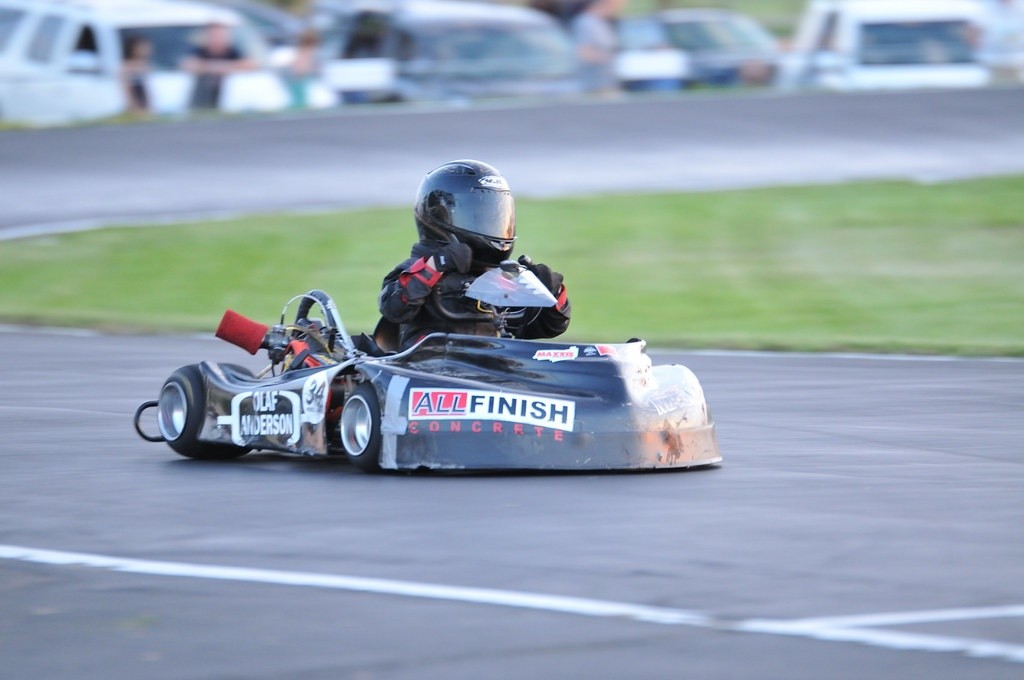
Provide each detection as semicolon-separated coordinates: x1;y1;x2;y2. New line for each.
294;0;619;105
0;0;297;128
775;0;1005;93
567;8;785;93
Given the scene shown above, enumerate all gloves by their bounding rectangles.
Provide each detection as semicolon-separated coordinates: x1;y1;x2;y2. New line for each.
432;243;472;275
519;254;563;296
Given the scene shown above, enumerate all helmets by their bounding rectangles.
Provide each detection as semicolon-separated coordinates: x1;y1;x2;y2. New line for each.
414;159;515;277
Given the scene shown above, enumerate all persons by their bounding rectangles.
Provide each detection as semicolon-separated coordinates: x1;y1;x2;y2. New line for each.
115;36;156;117
570;0;624;102
182;23;258;111
378;158;572;353
